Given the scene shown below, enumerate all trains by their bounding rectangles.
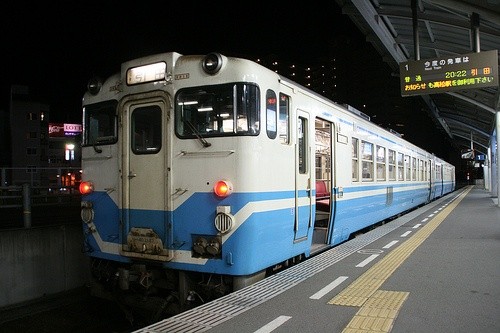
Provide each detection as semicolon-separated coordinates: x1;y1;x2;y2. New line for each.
78;51;456;300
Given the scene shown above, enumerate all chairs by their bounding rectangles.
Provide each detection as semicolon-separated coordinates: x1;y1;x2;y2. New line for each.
315;181;330;213
